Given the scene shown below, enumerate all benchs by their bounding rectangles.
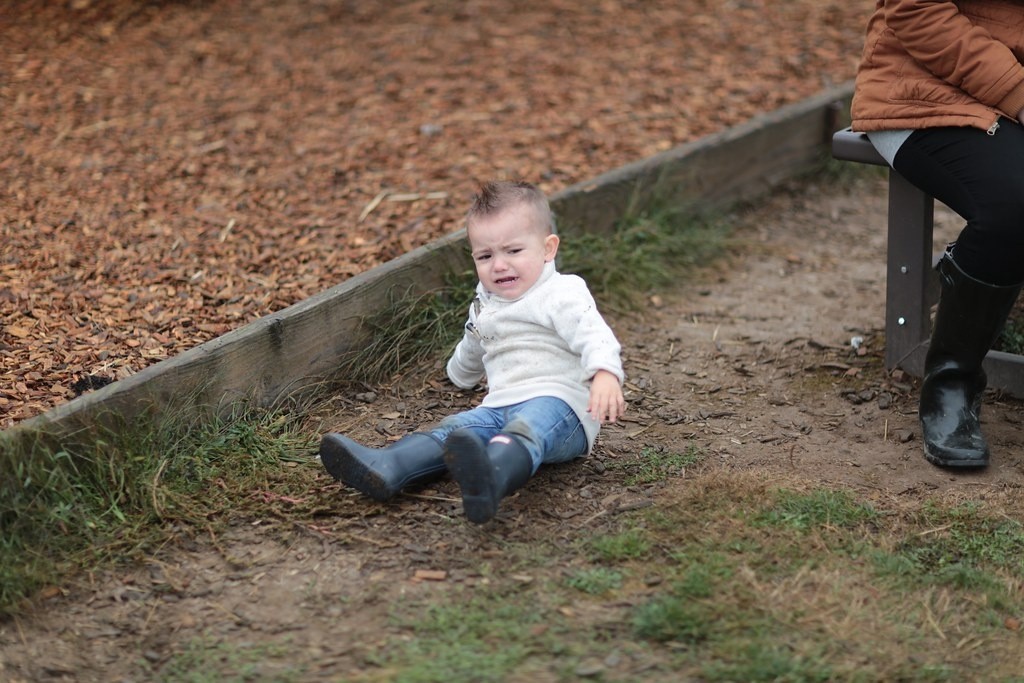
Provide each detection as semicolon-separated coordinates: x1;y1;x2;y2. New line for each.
830;126;1024;399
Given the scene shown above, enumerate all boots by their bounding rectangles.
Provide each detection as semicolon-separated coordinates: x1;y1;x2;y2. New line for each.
319;432;446;503
444;429;534;524
918;241;1020;468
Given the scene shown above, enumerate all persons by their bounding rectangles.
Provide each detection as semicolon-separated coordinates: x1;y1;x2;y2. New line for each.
849;0;1024;471
320;179;625;527
